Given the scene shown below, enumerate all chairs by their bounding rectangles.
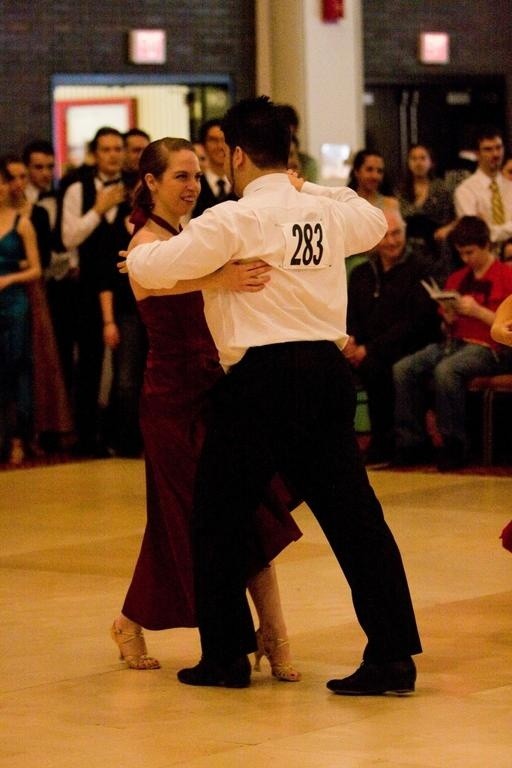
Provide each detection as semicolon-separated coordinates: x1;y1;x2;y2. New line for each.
425;374;512;466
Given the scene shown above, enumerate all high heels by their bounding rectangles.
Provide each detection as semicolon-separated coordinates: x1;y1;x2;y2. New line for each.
111;620;160;670
255;628;301;681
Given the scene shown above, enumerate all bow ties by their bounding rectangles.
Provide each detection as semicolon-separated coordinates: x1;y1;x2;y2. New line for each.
104;179;120;186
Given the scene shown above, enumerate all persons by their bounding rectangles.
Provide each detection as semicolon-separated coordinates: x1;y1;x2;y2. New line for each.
181;117;243;221
491;293;511;553
117;94;422;696
288;132;303;178
1;127;151;467
345;129;512;471
113;136;305;683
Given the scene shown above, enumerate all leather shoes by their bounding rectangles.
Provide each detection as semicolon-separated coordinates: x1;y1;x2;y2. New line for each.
326;664;416;694
178;663;251;688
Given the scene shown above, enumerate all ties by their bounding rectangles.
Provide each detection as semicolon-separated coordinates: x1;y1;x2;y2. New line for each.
489;181;504;225
218;179;226;198
